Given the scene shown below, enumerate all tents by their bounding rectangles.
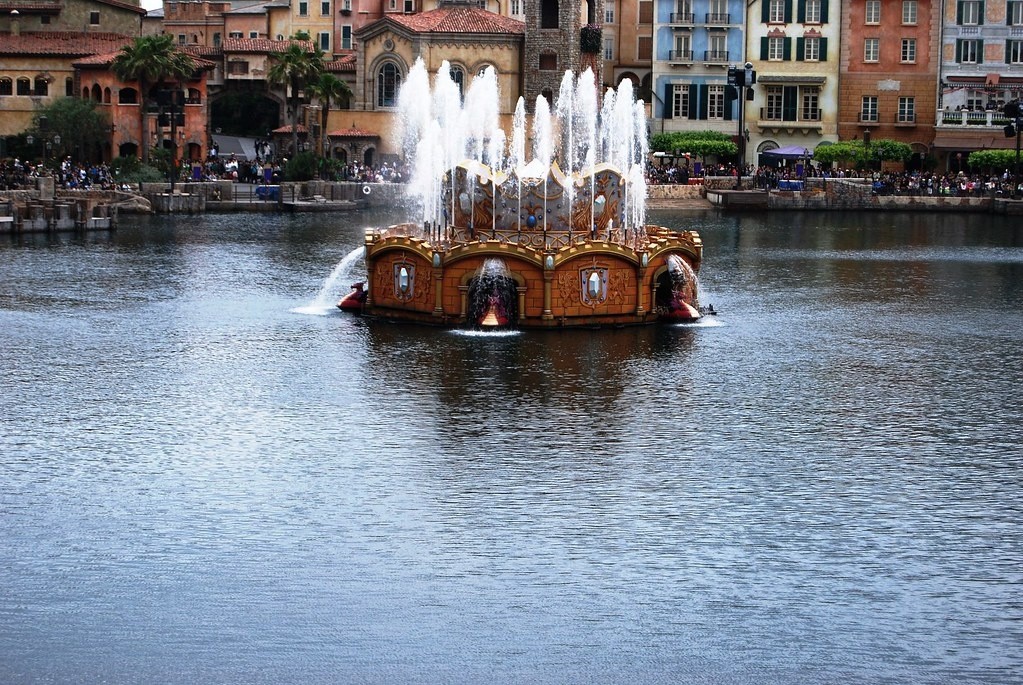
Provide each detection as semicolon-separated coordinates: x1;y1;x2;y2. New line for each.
762;145;814;159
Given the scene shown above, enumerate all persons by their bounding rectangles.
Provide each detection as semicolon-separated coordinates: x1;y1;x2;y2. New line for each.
945;105;966;111
0;137;422;193
646;163;1023;198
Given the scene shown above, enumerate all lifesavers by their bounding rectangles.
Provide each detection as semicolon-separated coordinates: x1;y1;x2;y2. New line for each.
362;186;371;194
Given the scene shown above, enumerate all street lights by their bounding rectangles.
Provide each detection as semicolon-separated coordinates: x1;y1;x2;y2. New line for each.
729;62;758;191
798;149;811;172
27;114;63;176
1003;100;1022;198
158;102;185;193
863;127;871;183
